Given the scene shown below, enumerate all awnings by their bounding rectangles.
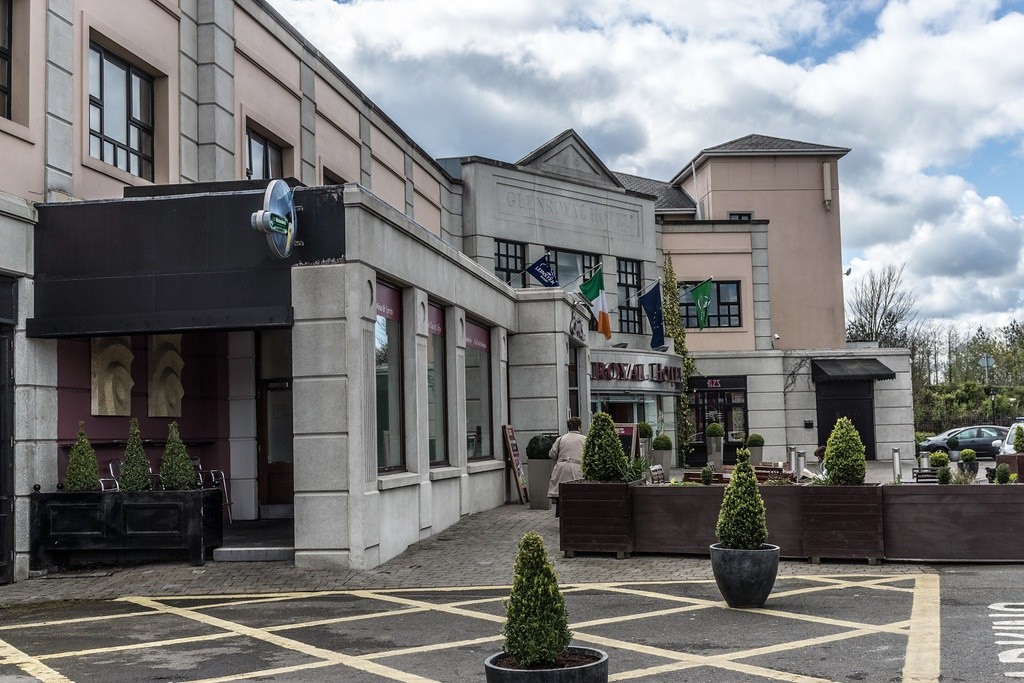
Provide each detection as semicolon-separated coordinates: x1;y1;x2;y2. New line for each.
812;358;896;384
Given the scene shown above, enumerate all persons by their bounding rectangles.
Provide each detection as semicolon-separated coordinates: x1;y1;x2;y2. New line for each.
548;418;587;517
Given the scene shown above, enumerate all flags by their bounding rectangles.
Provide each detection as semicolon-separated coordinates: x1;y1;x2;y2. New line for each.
579;267;611;341
525;253;559;287
691;279;712;331
639;281;664;348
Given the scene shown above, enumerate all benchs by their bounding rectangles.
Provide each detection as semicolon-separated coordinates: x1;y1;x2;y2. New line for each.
912;468;938;479
754;467;782;484
649;465;672;483
684;473;730;483
916;473;939;484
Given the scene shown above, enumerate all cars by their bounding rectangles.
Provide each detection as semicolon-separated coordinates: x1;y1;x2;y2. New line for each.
920;428;997;458
948;425;1009;457
687;432;705;443
992;423;1024;455
723;431;746;442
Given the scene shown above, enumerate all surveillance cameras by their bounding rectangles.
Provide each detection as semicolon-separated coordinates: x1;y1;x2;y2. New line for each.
774;333;780;339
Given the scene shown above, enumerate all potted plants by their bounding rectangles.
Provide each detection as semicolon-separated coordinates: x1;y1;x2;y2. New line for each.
747;434;765;466
28;418;224;571
638;421;652;457
996;425;1024;482
710;449;779;610
929;451;948;474
705;422;725;470
559;412;647;559
482;530;609;683
651;435;672;481
957;449;978;477
800;416;884;564
525;435;554;510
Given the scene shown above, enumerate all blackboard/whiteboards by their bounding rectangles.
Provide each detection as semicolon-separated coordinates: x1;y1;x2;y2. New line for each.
614;424;637;463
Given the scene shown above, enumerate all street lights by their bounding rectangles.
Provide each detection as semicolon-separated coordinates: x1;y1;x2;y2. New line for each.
989;389;997;424
